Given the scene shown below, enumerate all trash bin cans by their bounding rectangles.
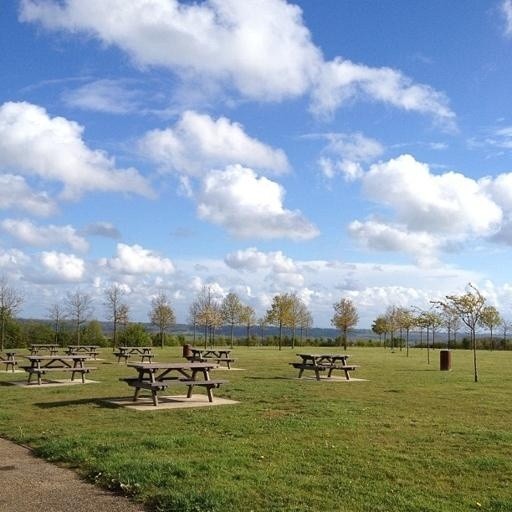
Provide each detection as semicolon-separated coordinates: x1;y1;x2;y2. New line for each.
440;350;452;371
183;344;192;357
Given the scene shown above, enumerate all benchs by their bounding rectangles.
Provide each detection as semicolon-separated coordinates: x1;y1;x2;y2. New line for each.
313;365;360;368
18;365;89;372
288;363;335;365
143;379;229;387
62;350;100;354
118;376;186;382
113;352;155;357
28;348;58;352
1;360;19;364
186;355;236;362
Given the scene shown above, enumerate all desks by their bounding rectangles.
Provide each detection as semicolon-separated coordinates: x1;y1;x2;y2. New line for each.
67;345;97;360
126;363;219;406
24;355;91;385
296;354;352;381
0;352;17;373
118;347;151;364
30;345;61;356
187;350;232;370
